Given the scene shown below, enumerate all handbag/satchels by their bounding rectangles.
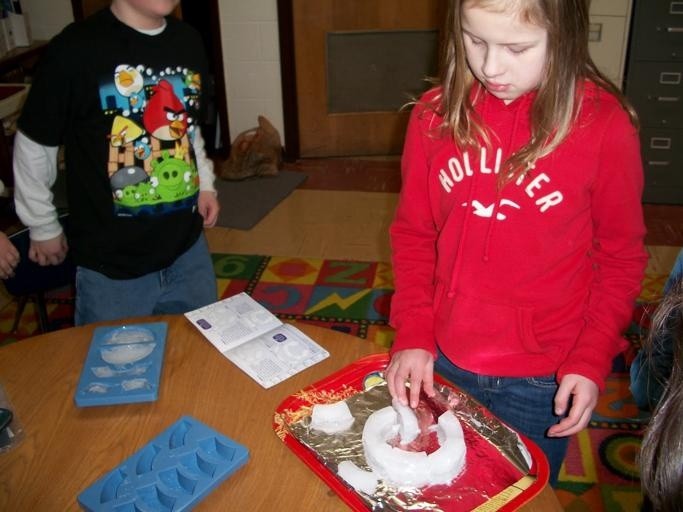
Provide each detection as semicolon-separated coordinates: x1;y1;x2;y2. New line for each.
220;115;283;181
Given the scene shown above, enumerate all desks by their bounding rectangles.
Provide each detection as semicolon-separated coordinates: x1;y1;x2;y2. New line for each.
0;312;567;512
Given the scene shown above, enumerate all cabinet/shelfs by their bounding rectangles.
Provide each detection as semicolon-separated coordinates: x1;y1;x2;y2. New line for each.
587;0;683;206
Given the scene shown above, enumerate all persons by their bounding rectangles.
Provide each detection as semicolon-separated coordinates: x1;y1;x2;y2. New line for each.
11;0;223;328
383;0;651;487
0;227;21;279
627;251;681;511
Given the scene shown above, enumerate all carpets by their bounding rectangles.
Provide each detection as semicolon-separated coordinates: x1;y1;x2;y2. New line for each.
2;250;668;511
214;169;309;230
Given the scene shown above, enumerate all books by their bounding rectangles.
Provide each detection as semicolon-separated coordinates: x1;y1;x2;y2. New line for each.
182;292;330;390
